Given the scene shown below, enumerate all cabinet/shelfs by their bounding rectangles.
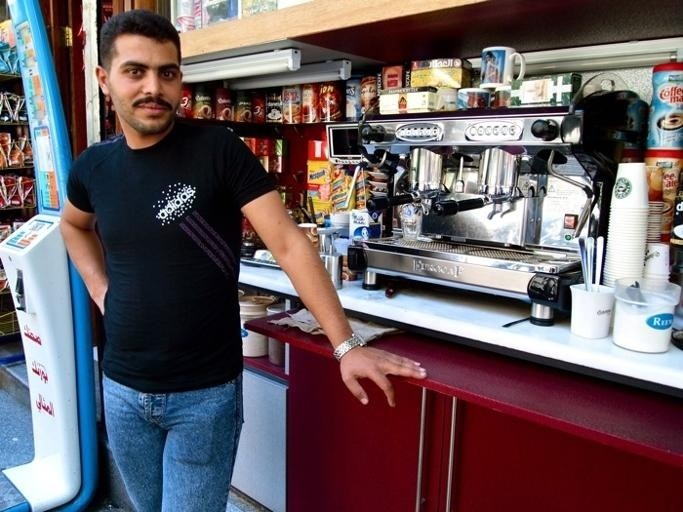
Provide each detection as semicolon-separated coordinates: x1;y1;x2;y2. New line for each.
0;71;41;345
242;305;683;512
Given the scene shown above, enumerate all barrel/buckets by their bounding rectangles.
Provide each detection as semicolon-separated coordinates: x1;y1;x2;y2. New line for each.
240;295;279;357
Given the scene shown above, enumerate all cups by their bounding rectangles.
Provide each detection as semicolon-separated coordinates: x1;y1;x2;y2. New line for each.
456;46;526;109
399;203;423;241
325;254;343;289
569;161;672;338
297;223;320;253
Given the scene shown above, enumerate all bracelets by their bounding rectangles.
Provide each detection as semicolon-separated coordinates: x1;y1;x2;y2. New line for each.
332;331;367;361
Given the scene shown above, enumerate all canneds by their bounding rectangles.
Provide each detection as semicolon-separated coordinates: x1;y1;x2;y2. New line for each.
176;74;381;124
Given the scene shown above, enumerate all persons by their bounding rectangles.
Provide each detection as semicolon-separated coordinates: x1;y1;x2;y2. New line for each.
57;11;428;512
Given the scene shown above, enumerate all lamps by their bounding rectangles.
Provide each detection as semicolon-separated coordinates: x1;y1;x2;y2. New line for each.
181;47;353;91
